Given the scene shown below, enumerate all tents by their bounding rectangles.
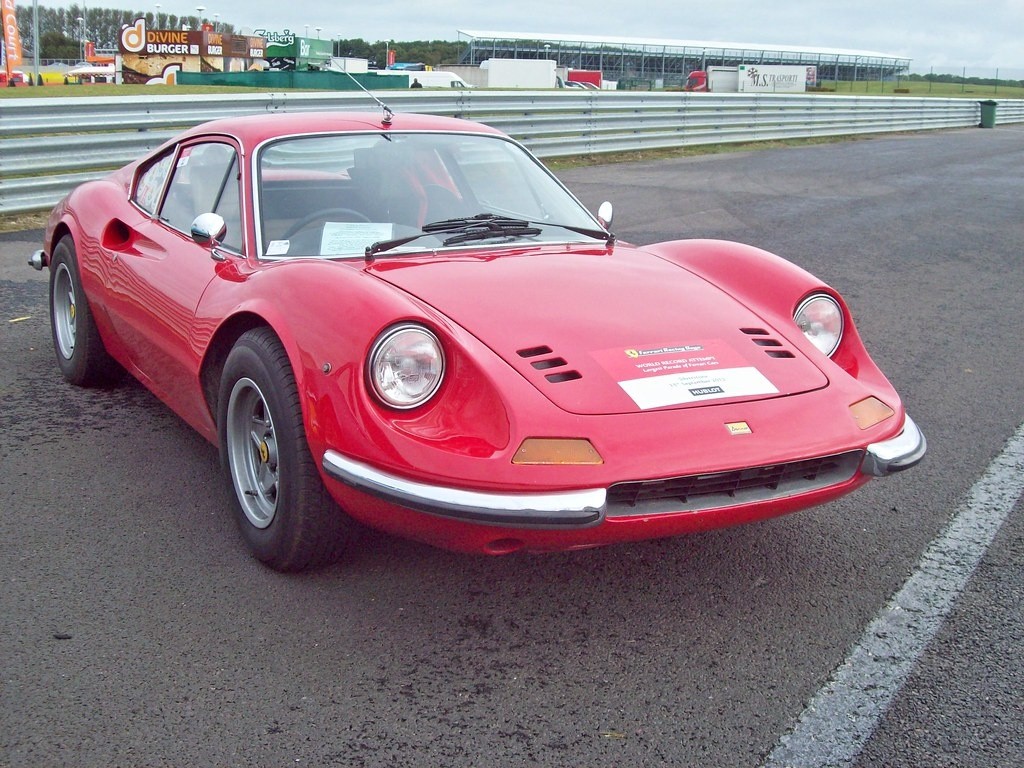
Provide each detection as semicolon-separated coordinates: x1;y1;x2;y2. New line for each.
64;66;117;83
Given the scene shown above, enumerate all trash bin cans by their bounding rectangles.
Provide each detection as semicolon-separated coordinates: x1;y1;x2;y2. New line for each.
977;100;996;128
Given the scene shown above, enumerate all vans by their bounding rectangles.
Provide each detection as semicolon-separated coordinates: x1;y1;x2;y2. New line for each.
378;70;473;88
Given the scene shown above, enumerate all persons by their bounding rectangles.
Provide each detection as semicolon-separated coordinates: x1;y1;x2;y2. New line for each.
409;78;422;88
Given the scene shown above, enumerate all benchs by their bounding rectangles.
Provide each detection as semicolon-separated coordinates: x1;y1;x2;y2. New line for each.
189;155;469;253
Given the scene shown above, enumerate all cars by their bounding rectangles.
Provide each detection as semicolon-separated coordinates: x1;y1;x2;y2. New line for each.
29;110;929;575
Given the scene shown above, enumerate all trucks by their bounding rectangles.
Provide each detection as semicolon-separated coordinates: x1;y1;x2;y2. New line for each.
686;66;738;93
557;64;604;88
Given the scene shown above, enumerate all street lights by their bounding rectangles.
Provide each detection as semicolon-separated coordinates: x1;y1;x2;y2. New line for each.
196;5;206;29
213;13;221;32
304;25;309;37
155;4;163;30
337;33;341;57
74;17;89;62
315;28;322;39
384;40;389;69
543;44;551;59
